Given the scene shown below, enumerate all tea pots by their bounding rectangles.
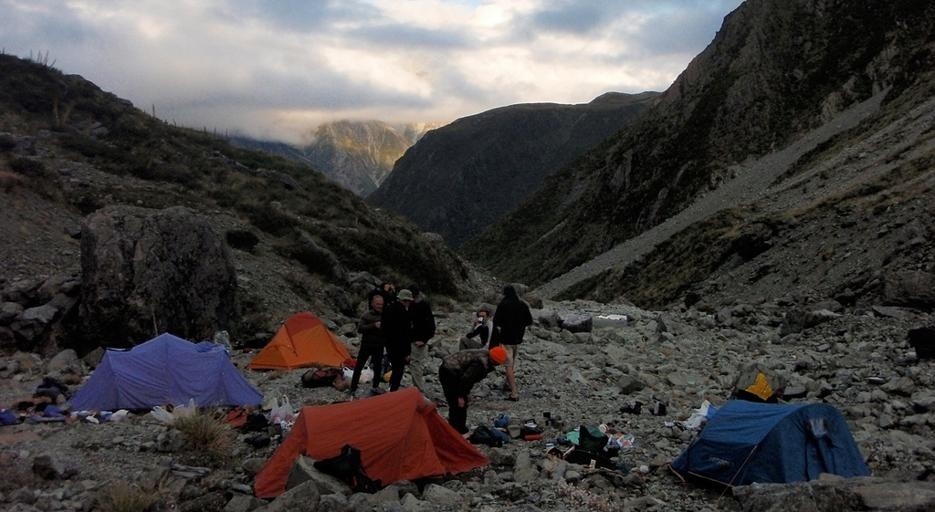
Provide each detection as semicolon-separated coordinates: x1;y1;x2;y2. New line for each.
524;418;538;431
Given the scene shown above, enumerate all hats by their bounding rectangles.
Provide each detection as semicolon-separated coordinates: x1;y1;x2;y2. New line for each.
396;289;414;301
489;347;508;364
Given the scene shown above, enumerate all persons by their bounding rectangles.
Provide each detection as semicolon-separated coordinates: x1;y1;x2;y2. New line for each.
350;282;436;394
438;344;509;434
459;308;493;350
489;284;533;401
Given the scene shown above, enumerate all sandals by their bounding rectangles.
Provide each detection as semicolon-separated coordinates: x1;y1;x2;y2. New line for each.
504;396;519;402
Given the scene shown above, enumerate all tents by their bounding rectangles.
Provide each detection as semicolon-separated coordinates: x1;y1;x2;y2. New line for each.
668;399;871;500
253;386;490;501
67;332;264;411
250;310;352;371
